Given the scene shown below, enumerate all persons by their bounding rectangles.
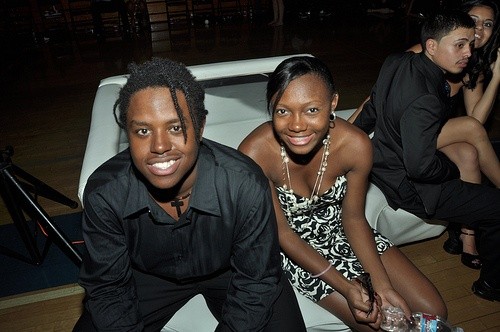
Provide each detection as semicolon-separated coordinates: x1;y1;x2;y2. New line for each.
346;0;500;269
353;5;499;301
236;55;447;332
269;0;284;26
74;57;309;332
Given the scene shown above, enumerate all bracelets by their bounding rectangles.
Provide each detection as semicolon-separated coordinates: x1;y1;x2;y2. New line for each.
310;261;333;278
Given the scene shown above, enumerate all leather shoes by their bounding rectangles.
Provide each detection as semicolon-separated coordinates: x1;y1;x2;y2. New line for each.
472;271;500;301
443;233;462;254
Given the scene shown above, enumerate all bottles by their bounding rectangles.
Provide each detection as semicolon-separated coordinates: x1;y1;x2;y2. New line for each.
380;306;464;332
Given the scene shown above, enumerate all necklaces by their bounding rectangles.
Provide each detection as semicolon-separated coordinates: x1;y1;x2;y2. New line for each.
280;129;332;228
154;189;193;218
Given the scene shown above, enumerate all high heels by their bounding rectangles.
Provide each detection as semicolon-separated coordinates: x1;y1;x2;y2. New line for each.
456;227;482;270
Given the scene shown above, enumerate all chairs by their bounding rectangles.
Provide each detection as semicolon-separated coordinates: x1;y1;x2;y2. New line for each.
78;54;351;332
